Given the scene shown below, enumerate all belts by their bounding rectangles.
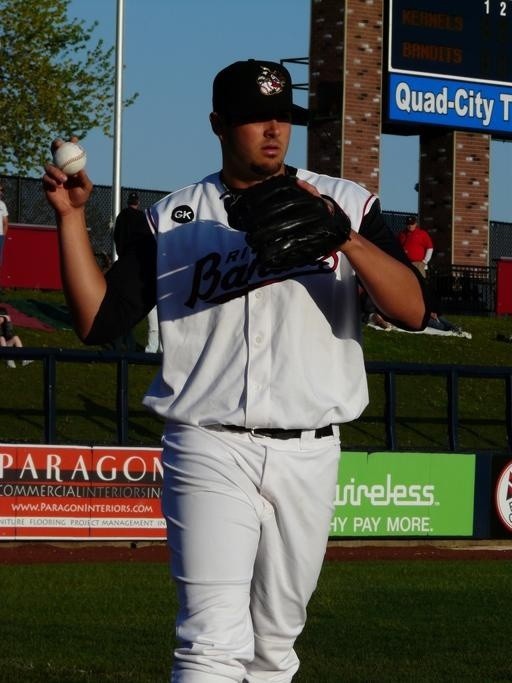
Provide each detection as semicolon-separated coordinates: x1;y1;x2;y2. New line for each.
223;419;334;441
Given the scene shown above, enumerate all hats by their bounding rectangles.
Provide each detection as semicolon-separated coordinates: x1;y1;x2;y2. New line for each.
213;60;321;125
407;216;416;225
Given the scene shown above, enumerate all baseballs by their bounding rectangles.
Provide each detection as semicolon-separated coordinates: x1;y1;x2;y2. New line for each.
55;141;86;175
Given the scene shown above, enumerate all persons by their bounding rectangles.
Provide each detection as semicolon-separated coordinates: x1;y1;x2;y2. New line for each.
0;308;36;369
0;185;11;261
369;299;466;335
39;56;432;682
113;191;143;260
397;214;433;280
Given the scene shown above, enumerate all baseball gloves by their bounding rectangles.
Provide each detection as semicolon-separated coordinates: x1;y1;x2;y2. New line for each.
219;173;351;268
